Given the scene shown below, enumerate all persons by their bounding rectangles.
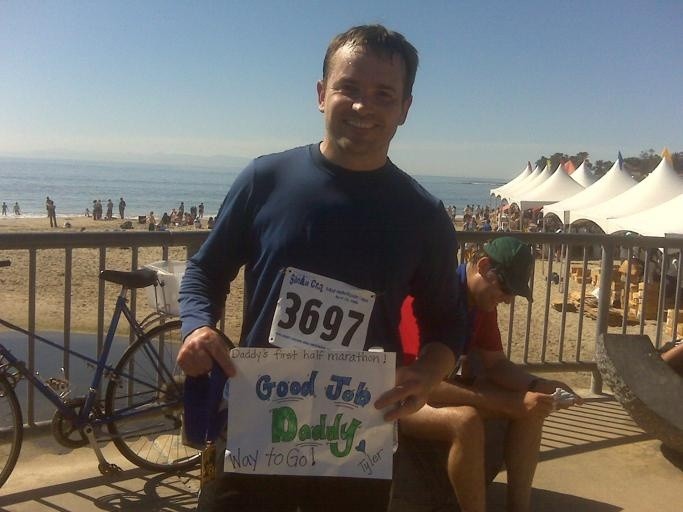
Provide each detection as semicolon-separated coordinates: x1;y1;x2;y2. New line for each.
446;203;509;262
0;202;9;215
398;235;585;511
657;338;683;375
45;196;217;231
13;202;20;215
175;23;466;511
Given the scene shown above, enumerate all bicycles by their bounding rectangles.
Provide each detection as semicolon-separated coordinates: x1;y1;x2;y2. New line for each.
0;258;235;488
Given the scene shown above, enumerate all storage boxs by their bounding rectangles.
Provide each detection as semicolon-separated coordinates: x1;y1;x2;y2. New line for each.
664;308;683;338
143;259;186;317
570;259;659;320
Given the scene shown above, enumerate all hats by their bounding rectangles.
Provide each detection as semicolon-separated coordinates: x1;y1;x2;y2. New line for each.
482;236;533;304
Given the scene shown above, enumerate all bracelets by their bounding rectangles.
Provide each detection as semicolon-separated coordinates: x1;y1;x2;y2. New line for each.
528;377;545;392
